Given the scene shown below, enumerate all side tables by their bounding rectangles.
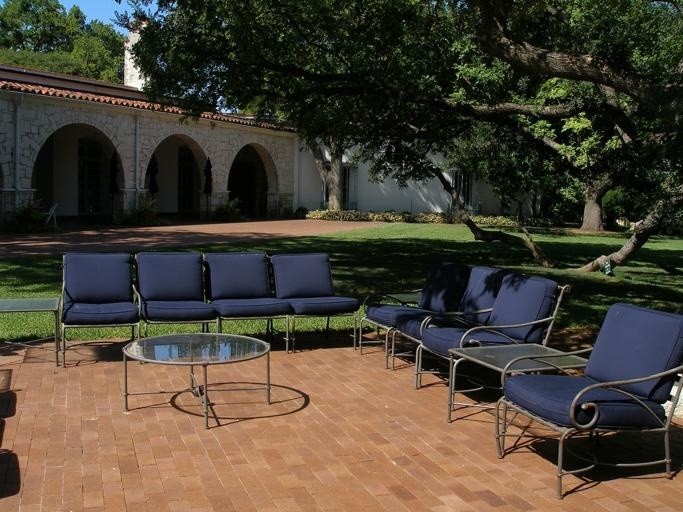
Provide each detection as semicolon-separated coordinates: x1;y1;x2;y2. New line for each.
0;297;62;366
447;344;588;456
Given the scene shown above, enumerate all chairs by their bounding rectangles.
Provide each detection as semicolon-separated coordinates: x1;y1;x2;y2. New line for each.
495;302;683;499
203;252;289;354
390;266;507;389
136;252;220;356
359;258;469;355
271;253;360;353
54;252;142;368
416;272;570;410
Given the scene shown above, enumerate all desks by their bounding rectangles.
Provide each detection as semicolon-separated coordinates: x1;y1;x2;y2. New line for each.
122;333;271;429
